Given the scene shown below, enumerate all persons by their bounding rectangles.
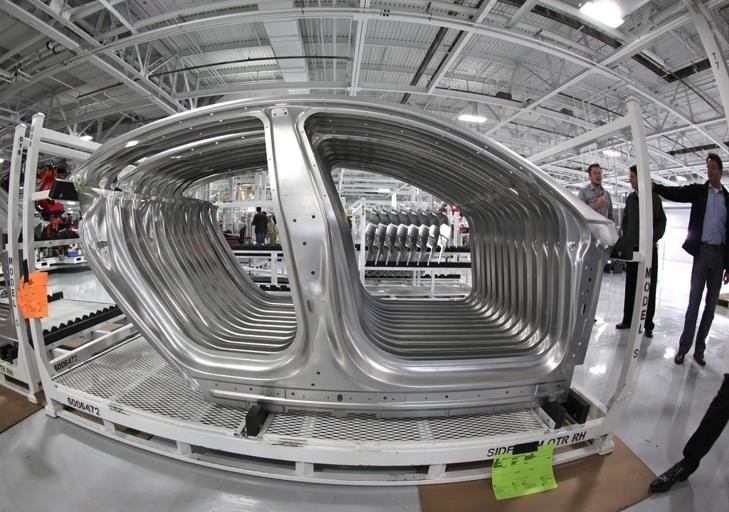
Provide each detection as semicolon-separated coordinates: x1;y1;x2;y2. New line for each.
238;207;277;248
579;162;614;324
614;165;668;340
645;371;729;493
651;153;728;367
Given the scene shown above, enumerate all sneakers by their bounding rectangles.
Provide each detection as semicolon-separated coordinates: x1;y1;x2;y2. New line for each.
645;329;653;338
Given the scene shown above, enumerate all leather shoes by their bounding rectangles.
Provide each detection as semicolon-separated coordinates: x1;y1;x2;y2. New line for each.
693;353;705;365
649;457;700;491
616;322;630;329
675;351;684;364
